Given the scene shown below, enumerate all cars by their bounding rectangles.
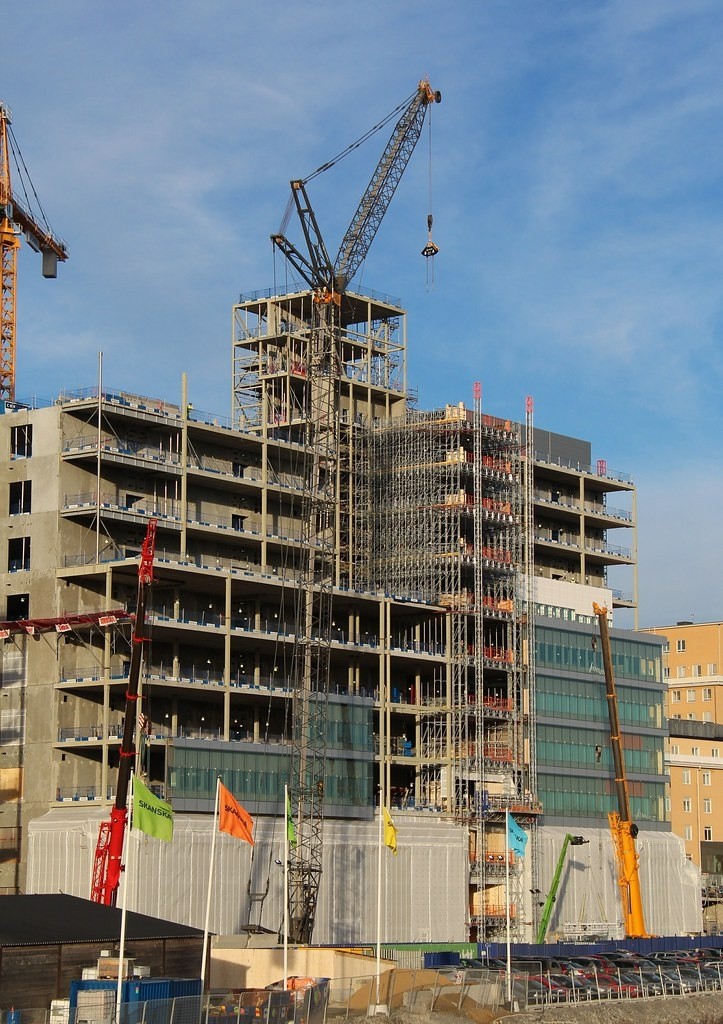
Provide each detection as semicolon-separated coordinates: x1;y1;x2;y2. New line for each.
426;948;723;1010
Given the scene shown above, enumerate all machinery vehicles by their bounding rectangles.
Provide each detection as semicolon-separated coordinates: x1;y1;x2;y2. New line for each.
89;517;162;910
592;601;662;940
537;833;590;950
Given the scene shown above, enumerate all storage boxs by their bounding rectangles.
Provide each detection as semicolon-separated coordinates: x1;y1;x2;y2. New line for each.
69;977;200;1024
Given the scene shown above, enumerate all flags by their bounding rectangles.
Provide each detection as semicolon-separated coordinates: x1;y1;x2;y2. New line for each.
383;807;399;856
506;811;527;857
219;781;255;845
287;793;297;850
132;774;174;843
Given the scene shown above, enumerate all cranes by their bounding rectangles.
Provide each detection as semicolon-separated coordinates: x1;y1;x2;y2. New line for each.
0;99;69;401
270;77;442;950
